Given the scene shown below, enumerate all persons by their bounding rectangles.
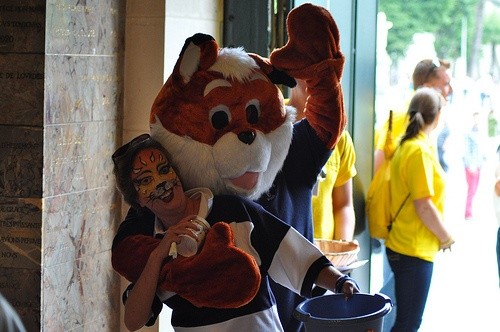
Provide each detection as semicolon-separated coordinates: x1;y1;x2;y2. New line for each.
284;79;356;243
112;134;360;332
374;58;452;332
386;88;456;332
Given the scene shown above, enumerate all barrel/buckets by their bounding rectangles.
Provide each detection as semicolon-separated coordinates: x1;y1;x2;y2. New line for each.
293;292;394;332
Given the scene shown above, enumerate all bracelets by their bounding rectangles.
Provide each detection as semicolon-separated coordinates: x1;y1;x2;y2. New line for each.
441;237;452;246
335;275;359;293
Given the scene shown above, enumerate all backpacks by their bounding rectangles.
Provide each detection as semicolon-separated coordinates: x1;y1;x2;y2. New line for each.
365;141;412;240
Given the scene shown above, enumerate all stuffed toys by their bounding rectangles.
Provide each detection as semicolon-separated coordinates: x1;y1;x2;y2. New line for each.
111;4;346;331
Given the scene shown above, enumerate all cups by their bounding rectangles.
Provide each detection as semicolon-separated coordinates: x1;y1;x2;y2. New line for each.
175;215;211;258
